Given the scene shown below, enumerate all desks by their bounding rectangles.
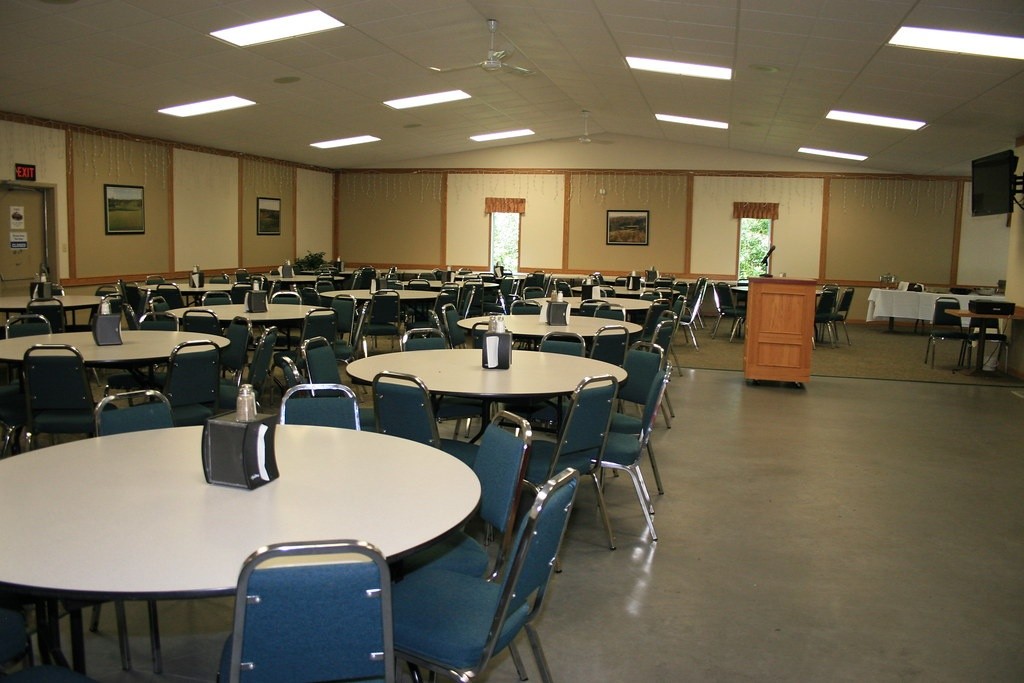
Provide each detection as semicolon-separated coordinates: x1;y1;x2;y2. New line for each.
395;281;499;298
944;301;1024;374
865;287;1005;333
729;285;828;340
259;275;346;292
0;423;482;683
522;297;661;321
320;289;450;321
457;314;643;353
0;296;105;332
569;285;681;301
165;303;334;353
138;283;250;304
346;348;629;444
450;272;527;281
0;330;230;405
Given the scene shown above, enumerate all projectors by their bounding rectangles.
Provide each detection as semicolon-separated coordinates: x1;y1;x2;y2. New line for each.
971;298;1016;315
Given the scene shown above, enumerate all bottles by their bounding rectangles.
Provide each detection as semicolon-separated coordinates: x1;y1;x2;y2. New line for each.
556;291;563;303
551;290;557;302
34;273;41;283
41;273;46;282
100;299;113;314
236;388;255;423
496;316;506;333
197;265;200;273
488;316;497;332
253;280;260;291
193;264;197;273
241;384;257;416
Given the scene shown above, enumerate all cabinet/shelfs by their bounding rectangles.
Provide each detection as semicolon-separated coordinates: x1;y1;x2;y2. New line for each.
744;275;817;389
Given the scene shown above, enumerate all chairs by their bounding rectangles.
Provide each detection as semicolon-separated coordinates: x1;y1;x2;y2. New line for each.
0;265;709;580
924;297;972;369
888;282;926;331
958;298;1010;373
218;538;396;683
387;468;581;683
712;272;854;349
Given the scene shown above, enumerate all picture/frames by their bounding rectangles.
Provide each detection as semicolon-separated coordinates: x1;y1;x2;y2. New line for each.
256;196;282;236
606;210;649;246
103;183;147;234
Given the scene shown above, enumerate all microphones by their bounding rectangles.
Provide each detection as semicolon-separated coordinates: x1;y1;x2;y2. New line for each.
761;245;776;264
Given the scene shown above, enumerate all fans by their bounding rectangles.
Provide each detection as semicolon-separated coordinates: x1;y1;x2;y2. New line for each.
442;18;534;74
554;110;613;144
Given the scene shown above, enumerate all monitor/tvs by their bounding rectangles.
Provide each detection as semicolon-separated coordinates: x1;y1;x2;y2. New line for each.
971;150;1014;216
